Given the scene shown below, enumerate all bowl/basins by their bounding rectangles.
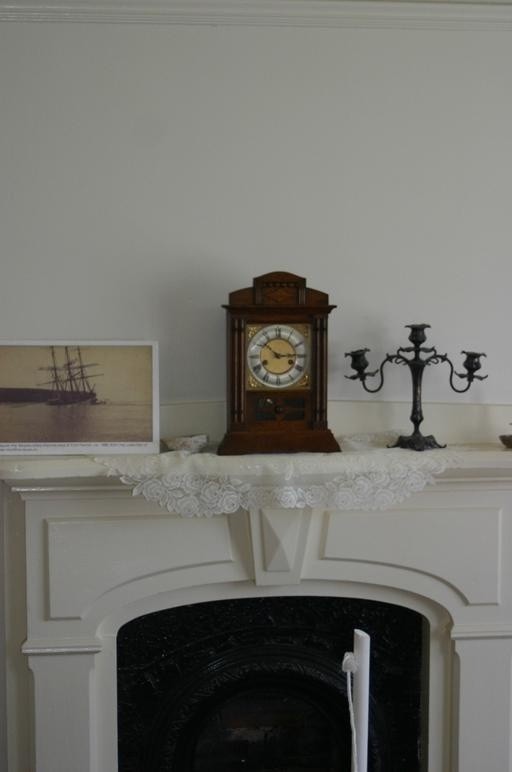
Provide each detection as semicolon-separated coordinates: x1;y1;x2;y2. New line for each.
499;435;512;448
160;434;207;453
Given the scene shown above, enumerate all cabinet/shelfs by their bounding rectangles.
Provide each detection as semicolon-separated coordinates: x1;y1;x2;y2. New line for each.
0;430;512;772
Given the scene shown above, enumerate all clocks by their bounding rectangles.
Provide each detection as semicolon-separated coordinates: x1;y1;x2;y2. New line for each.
216;267;347;455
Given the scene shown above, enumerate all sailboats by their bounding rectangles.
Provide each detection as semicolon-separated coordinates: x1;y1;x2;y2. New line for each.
0;346;106;404
